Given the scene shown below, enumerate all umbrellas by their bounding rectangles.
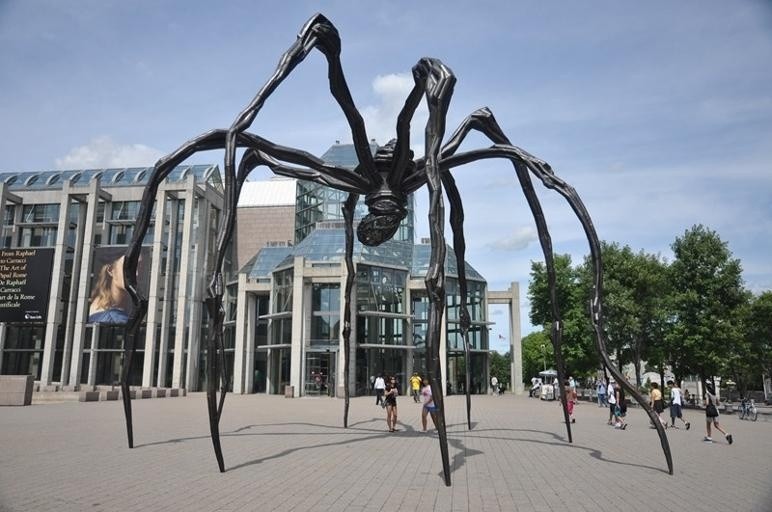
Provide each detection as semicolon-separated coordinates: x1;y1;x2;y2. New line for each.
539;366;557;377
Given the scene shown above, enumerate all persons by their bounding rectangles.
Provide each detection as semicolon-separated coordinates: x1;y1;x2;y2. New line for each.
491;375;498;396
408;371;422;403
529;376;732;444
420;377;438;434
87;247;142;325
374;373;386;405
384;376;402;432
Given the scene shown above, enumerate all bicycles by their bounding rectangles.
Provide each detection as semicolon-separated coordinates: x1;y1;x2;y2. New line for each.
736;396;757;422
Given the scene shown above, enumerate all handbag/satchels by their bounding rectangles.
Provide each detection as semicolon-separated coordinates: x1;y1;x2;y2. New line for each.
381;400;387;407
680;395;688;406
705;393;719;417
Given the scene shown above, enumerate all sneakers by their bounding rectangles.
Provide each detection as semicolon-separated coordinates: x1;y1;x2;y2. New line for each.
701;437;714;443
563;418;575;423
608;419;628;430
649;420;691;430
419;429;438;433
726;433;734;445
389;428;398;432
